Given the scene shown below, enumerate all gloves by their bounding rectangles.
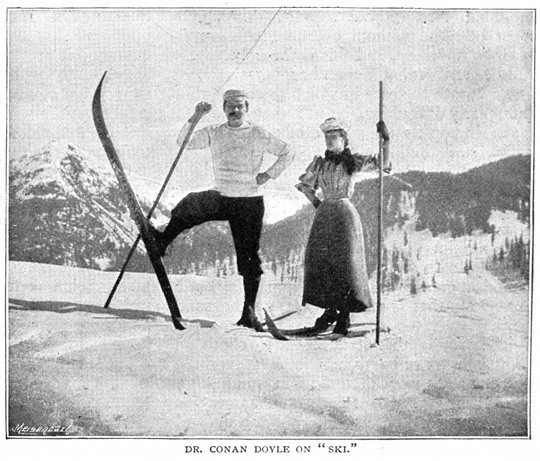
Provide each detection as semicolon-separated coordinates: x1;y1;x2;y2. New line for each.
312;197;322;208
376;119;389;140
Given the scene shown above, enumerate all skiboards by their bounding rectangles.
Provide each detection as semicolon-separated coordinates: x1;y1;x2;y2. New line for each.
90;68;299;330
262;308;392;341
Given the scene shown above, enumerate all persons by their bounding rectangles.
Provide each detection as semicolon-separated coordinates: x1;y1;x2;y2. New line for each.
295;117;392;336
147;89;294;329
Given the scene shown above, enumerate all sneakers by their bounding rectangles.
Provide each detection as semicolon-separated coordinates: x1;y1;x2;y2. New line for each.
314;308;339;331
332;312;351;336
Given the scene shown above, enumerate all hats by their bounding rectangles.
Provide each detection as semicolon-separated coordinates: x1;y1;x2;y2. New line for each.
222;88;250;102
319;115;352;133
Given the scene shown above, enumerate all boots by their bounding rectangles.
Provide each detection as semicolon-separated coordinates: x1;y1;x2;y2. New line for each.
146;213;187;255
236;278;267;332
